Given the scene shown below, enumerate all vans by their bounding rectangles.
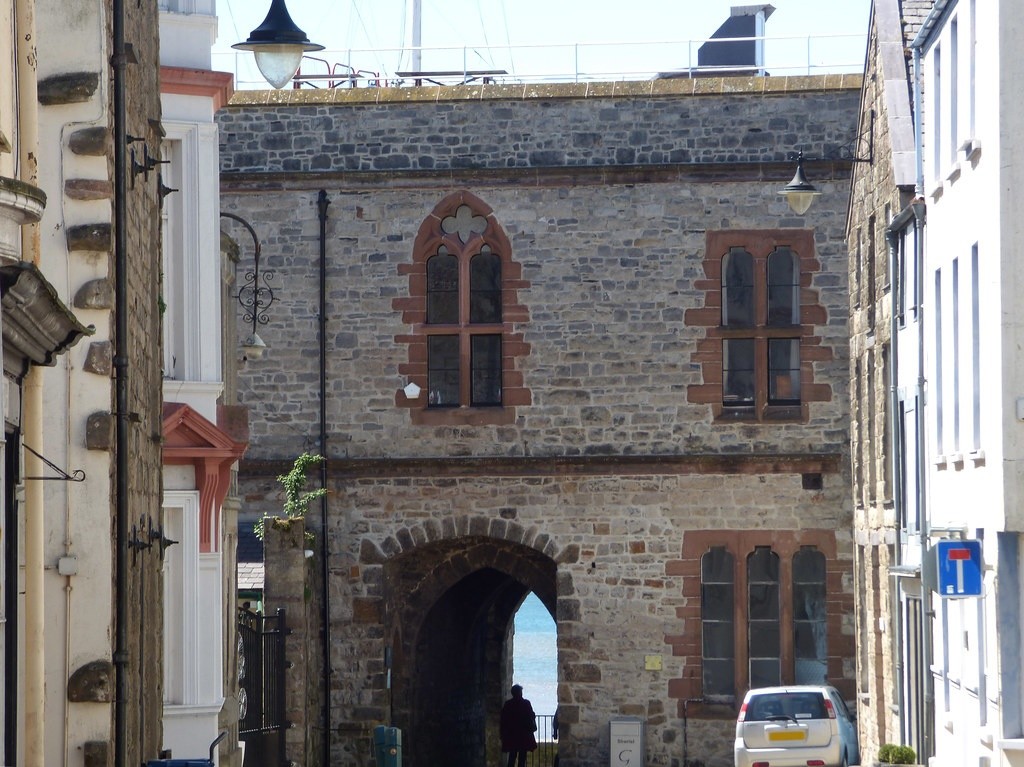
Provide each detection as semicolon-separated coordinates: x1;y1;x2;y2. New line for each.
733;685;858;767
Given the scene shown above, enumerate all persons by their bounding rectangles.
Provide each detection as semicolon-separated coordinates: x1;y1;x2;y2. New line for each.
552;711;560;767
498;685;538;767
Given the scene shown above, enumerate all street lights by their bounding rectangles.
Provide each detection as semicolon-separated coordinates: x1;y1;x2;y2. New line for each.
106;0;324;766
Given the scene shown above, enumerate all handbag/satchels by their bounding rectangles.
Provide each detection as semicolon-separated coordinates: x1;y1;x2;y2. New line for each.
523;699;537;733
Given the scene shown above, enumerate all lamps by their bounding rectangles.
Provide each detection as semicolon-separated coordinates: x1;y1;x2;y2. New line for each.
231;0;326;89
776;144;873;215
220;211;281;361
